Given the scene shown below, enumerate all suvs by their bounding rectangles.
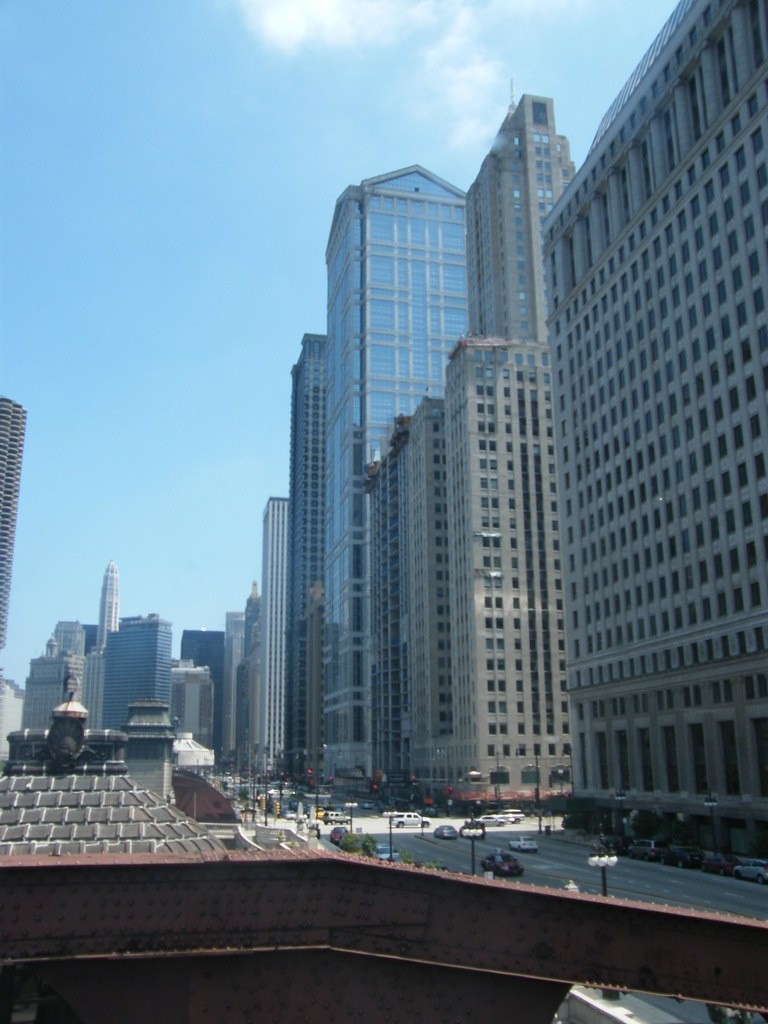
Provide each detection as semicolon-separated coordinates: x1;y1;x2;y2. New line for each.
491;809;525;824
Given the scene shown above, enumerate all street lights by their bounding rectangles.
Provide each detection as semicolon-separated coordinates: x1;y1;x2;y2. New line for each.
615;787;626;835
588;823;619;896
704;787;720;854
345;793;358;833
558;748;575;796
315;736;344;819
461;812;483;874
382;800;398;861
515;743;543;833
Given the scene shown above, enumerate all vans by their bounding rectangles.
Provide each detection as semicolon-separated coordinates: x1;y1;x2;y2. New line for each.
390;812;432;828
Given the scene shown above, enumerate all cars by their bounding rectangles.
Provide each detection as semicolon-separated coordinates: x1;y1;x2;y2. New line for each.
330;827;349;845
475;815;506;827
606;835;768;884
481;848;524;877
434;825;459;839
508;836;539;853
374;844;399;861
268;788;352;825
